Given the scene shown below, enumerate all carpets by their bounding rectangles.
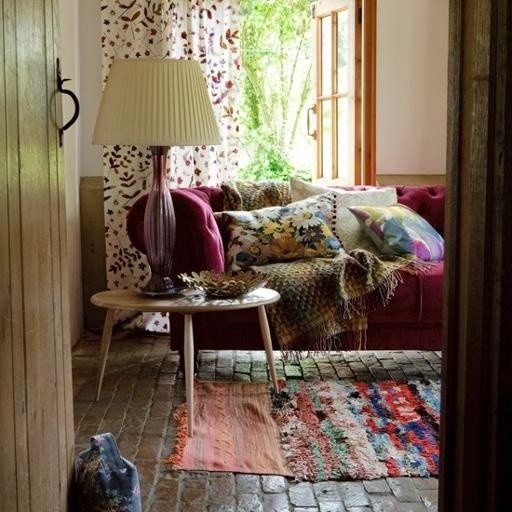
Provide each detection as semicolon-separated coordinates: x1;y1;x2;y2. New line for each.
269;374;440;483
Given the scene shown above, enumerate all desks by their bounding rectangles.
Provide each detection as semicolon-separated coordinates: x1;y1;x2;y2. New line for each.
88;280;282;438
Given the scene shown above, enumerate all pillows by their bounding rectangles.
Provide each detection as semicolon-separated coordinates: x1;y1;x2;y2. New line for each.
211;175;446;279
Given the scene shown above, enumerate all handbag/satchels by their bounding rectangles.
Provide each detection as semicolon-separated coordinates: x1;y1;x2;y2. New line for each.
69;431;143;512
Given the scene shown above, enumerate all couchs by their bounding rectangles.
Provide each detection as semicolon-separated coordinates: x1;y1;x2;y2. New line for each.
125;181;447;385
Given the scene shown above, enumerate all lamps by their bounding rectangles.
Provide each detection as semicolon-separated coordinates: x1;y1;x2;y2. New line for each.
90;59;225;299
